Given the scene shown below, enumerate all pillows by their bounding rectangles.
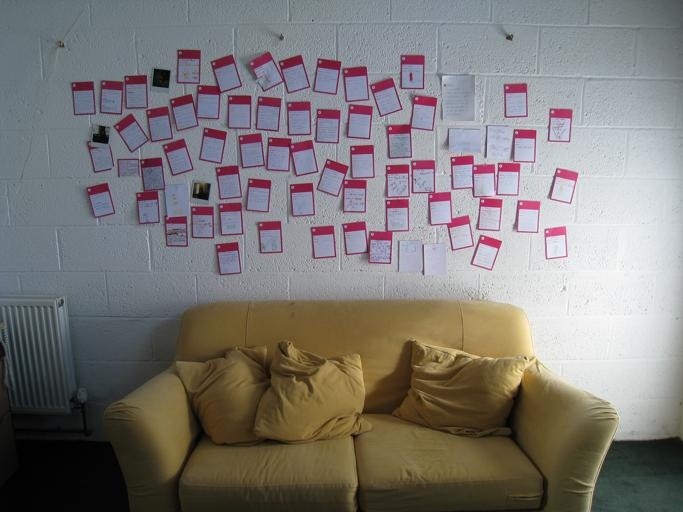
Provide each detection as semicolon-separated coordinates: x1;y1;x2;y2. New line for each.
169;343;276;448
249;339;375;444
393;337;538;439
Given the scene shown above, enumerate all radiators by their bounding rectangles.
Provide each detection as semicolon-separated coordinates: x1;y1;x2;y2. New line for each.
0;293;95;436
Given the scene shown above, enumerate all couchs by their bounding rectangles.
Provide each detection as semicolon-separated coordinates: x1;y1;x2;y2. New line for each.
105;300;620;512
